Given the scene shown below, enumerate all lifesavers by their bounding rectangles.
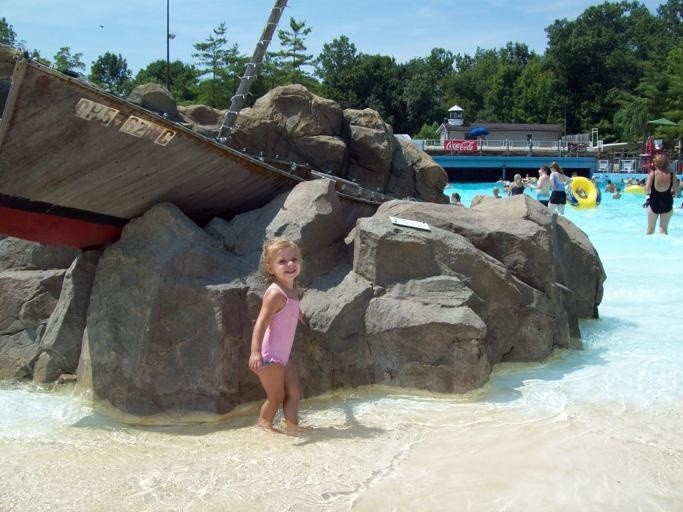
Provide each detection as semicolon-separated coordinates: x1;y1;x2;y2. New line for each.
564;176;601;209
624;185;644;194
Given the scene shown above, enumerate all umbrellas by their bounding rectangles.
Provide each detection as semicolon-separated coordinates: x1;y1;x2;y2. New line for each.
648;117;680;130
468;128;490;139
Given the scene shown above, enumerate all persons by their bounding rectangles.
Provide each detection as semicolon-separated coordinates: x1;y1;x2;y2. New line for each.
246;236;312;434
449;193;464;207
645;133;654;155
642;154;678;235
491;161;683;215
